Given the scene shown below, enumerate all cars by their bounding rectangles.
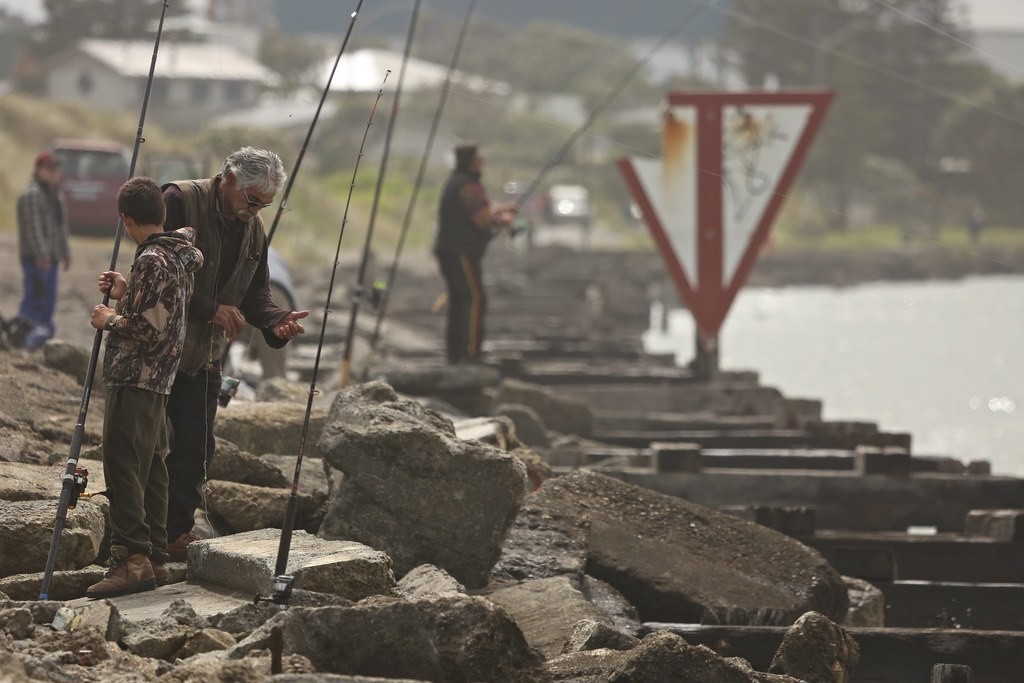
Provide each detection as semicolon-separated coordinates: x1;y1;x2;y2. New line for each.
542;184;594;229
53;136;143;241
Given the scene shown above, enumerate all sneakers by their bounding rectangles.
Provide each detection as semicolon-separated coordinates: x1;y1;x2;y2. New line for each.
152;562;169;584
167;532;202;561
86;545;156;598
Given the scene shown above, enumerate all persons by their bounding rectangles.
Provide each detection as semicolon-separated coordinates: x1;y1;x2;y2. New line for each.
159;147;309;559
17;155;73;339
88;176;203;599
432;146;517;366
223;248;298;378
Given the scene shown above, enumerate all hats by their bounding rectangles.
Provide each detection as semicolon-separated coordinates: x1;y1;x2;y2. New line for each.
36;153;57;165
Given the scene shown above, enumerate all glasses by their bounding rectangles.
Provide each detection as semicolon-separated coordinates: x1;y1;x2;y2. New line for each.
238;176;273;208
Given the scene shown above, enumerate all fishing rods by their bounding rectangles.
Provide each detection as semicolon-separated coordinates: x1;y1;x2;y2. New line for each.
37;0;168;600
216;1;363;409
253;68;391;605
428;0;711;316
362;1;477;389
338;2;421;390
